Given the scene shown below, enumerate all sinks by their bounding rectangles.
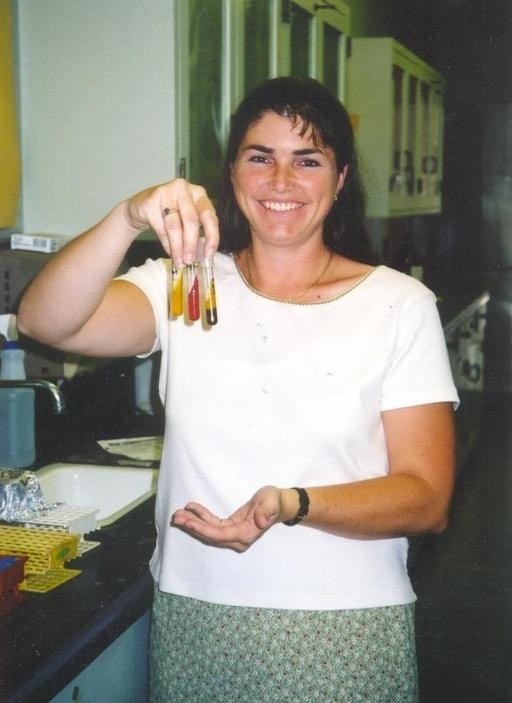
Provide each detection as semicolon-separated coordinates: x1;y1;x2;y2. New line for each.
31;462;159;530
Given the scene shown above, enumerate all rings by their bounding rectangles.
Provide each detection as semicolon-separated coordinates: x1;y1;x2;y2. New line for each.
162;208;178;218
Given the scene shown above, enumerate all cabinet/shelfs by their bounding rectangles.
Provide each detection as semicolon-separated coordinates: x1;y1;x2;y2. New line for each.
437;287;492;392
14;578;152;703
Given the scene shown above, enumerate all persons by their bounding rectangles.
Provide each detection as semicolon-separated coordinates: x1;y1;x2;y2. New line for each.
17;74;461;703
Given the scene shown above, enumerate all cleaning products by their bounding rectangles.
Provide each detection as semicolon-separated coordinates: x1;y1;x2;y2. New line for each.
0;313;37;470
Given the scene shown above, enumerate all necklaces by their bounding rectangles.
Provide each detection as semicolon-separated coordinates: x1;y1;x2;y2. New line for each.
246;247;334;302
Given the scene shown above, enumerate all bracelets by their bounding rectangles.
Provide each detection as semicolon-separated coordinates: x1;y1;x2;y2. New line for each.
283;488;310;526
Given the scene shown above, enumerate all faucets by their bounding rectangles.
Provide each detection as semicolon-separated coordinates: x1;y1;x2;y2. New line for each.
0;378;68;417
